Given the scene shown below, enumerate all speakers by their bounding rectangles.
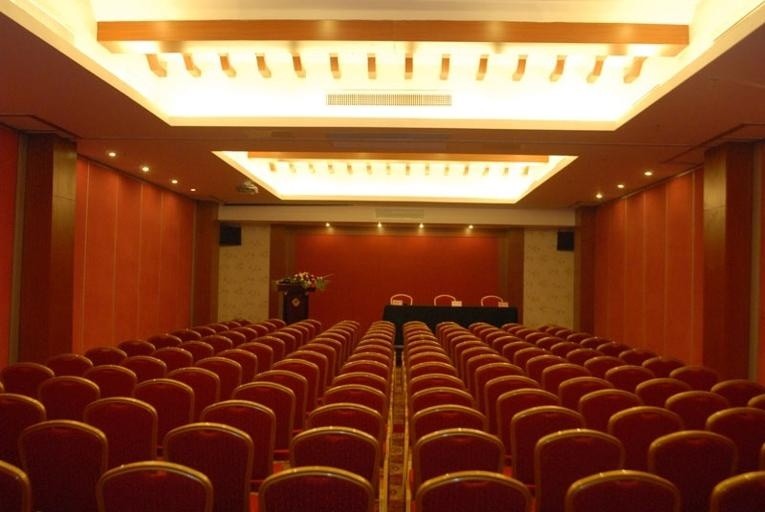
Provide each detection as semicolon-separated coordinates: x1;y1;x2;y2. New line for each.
221;224;241;245
557;232;573;250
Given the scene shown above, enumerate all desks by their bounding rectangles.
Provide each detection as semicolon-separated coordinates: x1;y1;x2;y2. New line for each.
383;305;518;367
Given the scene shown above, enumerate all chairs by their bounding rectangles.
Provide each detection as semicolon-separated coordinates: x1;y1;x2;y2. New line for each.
484;375;543;423
232;319;256;327
0;392;46;473
410;470;532;512
485;331;514;347
580;337;611;351
370;324;395;331
206;324;229;334
578;389;645;434
191;326;216;338
403;321;427;327
236;342;273;374
407;373;466;397
706;406;765;475
117;338;155;358
619;348;658;367
341;360;391;383
118;355;166;383
132;379;195;456
405;330;434;340
538;324;560;333
354;344;393;368
489;388;564;456
438;324;460;344
605;365;655;393
669;365;723;392
564;469;684;511
406;361;459;381
169;328;201;344
150;346;193;374
44;353;95;377
256;322;277;333
407;386;474;418
308;338;342;376
491;336;524;356
525;332;554;345
286;350;328;403
298;343;336;388
324;329;351;356
230;327;257;343
665;390;731;431
265;332;296;355
710;471;765;512
287;325;309;345
407;428;504;497
339;320;361;338
0;362;55;402
38;375;100;424
277;328;303;348
82;396;164;470
357;338;394;355
550;342;586;359
231;382;304;460
536;337;565;352
406;351;453;370
390;294;413;306
473;325;495;337
199;335;233;355
217;331;246;349
195;356;242;402
508;326;529;336
270;358;323;416
266;320;286;330
258;466;378;512
480;328;504;343
334;323;357;344
466;353;511;396
316;333;346;362
513;347;553;372
162;422;259;511
365;330;394;339
322;384;389;424
436;322;457;338
597;341;633;358
760;441;765;470
524;427;626;511
649;430;740;512
503;341;540;364
556;329;581;340
445;331;472;352
303;319;322;336
84;344;127;368
368;327;394;335
641;356;687;378
433;295;456;306
501;324;521;332
711;379;765;408
0;460;32;512
218;321;241;330
146;333;183;351
558;376;615;412
450;335;482;361
290;426;383;497
474;362;525;413
516;328;541;339
330;326;354;349
455;341;490;376
245;324;268;337
176;341;214;365
294;323;315;339
17;419;109;512
406;345;448;359
542;363;592;397
331;372;390;400
307;403;386;459
405;334;440;346
481;295;504;307
468;323;488;332
566;347;604;367
442;326;469;348
409;403;491;451
404;327;432;336
349;352;393;375
252;370;310;434
503;405;587;485
526;355;569;385
406;340;442;352
608;405;685;473
545;327;567;336
81;364;138;400
167;368;220;424
372;321;395;327
215;349;258;385
567;333;594;345
403;324;428;330
250;336;286;363
361;333;394;345
460;346;500;384
748;394;765;410
200;400;289;491
96;461;214;512
635;378;692;409
584;356;627;380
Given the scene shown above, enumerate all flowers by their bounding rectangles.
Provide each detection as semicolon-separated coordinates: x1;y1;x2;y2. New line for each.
275;271;336;293
